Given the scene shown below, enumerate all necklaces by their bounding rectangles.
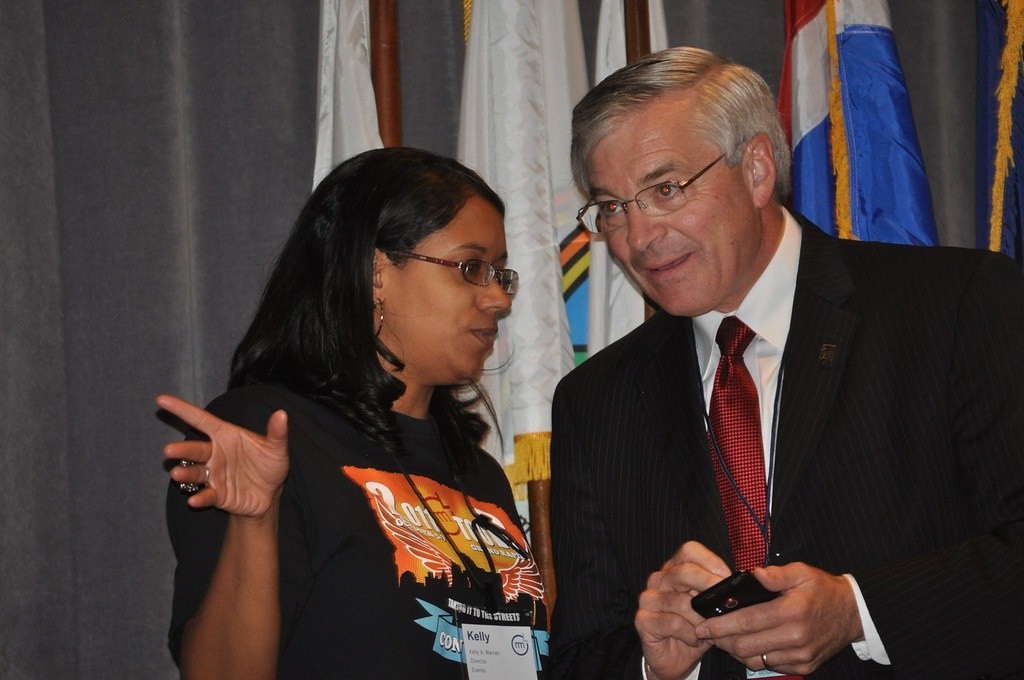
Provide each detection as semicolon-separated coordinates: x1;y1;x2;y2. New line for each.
697;365;784;556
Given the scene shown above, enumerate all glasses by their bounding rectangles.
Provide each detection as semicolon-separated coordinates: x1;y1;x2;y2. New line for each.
576;137;747;233
384;247;519;296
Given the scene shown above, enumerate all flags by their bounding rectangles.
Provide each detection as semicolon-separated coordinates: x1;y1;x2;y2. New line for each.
314;0;1023;598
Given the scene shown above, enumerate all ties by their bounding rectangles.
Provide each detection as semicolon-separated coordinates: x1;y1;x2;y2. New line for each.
705;316;768;576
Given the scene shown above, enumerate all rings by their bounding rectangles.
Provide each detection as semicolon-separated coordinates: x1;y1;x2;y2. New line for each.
181;460;209;491
762;654;771;670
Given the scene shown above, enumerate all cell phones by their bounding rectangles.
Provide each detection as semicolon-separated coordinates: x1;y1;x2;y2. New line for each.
690;569;778;620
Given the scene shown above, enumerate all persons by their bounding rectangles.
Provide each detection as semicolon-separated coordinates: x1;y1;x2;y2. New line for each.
156;145;549;680
546;47;1024;680
